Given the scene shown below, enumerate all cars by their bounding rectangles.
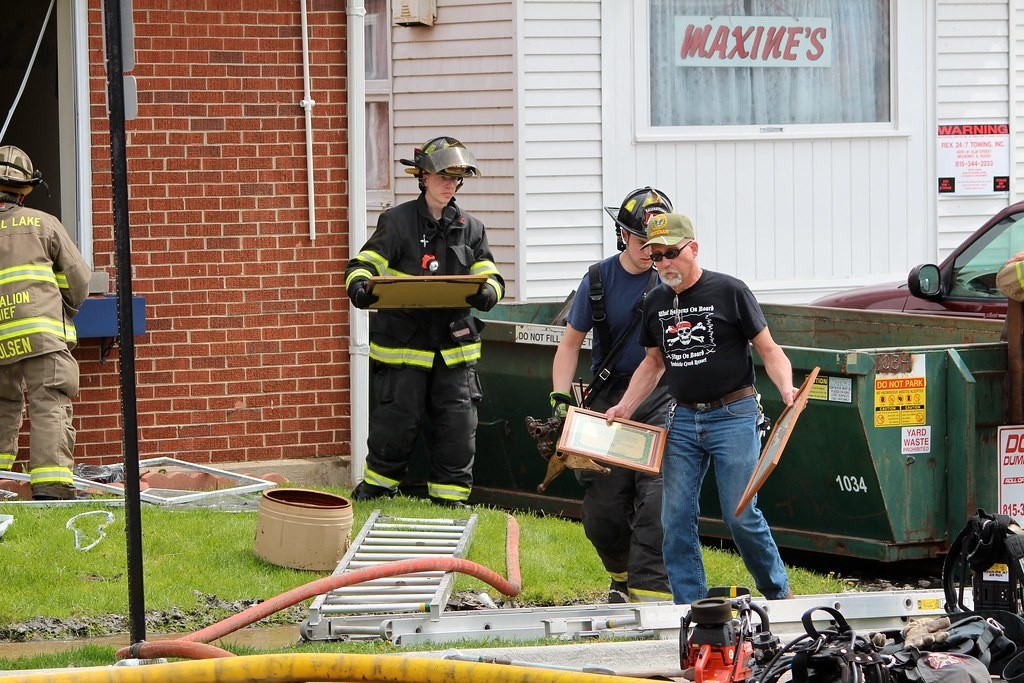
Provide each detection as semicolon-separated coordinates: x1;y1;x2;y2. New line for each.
795;201;1024;318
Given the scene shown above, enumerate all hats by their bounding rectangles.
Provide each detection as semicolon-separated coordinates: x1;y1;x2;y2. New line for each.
640;213;695;251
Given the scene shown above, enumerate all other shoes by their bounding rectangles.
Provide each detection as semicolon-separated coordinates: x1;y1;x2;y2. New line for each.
608;578;628;603
431;498;471;510
32;487;77;498
351;480;396;500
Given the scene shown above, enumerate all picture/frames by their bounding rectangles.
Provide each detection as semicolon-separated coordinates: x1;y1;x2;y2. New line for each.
734;366;821;518
558;405;668;475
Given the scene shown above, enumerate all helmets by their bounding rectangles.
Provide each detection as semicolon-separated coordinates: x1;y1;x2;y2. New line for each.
0;146;42;187
616;186;674;237
413;136;476;177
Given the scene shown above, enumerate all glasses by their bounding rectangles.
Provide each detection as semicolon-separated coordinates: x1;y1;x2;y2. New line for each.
649;241;691;262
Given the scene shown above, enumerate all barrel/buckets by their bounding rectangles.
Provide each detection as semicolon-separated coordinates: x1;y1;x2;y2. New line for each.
254;488;355;571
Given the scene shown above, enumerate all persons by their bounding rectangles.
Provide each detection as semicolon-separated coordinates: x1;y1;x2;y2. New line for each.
345;137;505;506
549;187;810;607
0;146;91;501
997;249;1024;427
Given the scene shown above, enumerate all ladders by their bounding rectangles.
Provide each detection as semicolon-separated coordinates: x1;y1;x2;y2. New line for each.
302;586;974;646
308;508;478;613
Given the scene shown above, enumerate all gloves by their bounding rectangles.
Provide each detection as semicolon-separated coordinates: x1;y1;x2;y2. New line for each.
549;391;572;420
466;284;498;312
348;277;379;309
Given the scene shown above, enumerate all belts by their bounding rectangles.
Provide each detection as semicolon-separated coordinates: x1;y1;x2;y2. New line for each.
678;385;756;411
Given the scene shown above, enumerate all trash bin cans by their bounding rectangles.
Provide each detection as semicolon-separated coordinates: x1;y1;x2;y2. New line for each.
373;301;1024;563
